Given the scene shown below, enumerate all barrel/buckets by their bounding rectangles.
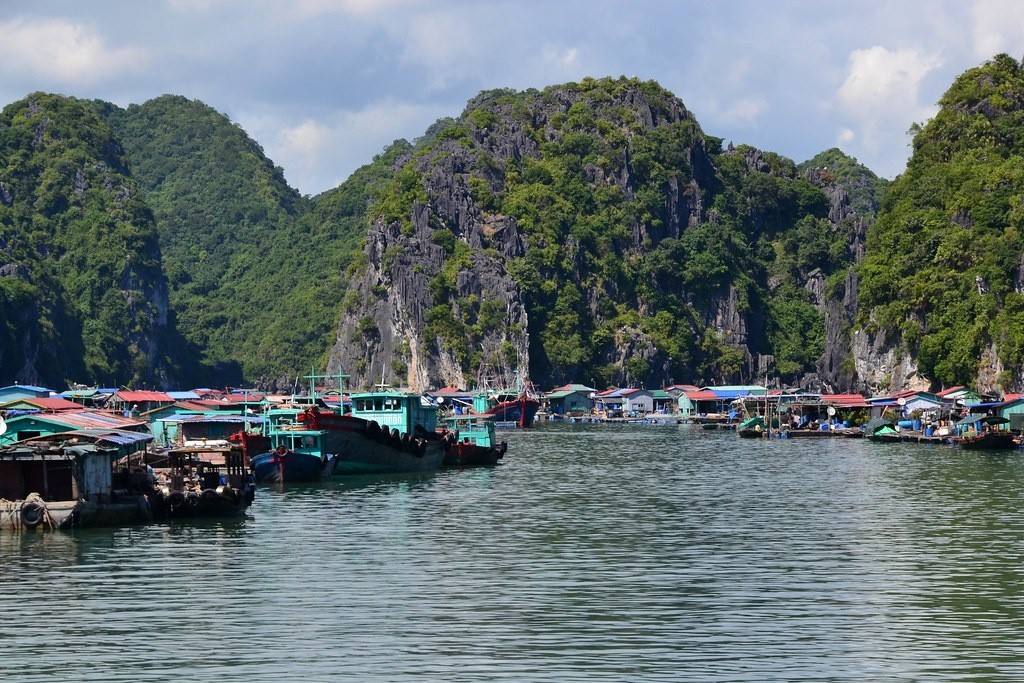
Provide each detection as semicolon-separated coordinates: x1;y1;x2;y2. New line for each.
659;405;663;410
843;420;850;428
803;415;808;422
220;474;227;486
912;420;921;431
926;428;933;437
184;491;189;498
898;420;912;428
977;420;981;430
599;404;603;409
701;413;705;417
963;423;967;432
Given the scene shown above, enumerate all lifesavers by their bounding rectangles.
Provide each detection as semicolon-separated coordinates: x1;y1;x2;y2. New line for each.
167;489;228;511
20;504;46;528
366;421;428;457
276;445;288;457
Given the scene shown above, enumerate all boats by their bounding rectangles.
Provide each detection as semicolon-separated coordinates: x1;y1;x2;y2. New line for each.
0;365;541;529
536;373;1024;448
